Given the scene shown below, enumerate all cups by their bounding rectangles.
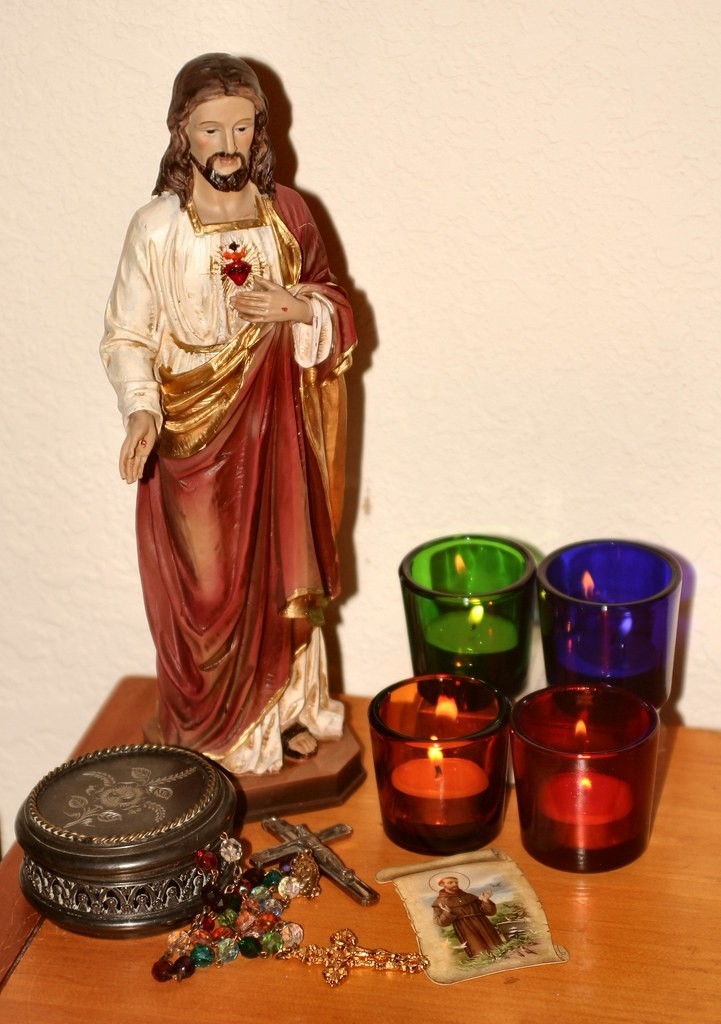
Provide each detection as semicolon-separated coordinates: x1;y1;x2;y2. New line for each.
368;673;512;856
537;539;682;721
510;683;660;873
399;533;536;713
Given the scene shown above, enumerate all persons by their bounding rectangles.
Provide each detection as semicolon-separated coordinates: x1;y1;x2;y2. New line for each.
101;52;359;775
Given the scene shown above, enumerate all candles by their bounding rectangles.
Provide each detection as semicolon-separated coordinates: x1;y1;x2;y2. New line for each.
510;680;662;873
398;532;538;708
534;537;682;706
367;674;513;852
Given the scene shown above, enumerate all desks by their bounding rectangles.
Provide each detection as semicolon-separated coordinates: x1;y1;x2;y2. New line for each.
0;675;721;1024
